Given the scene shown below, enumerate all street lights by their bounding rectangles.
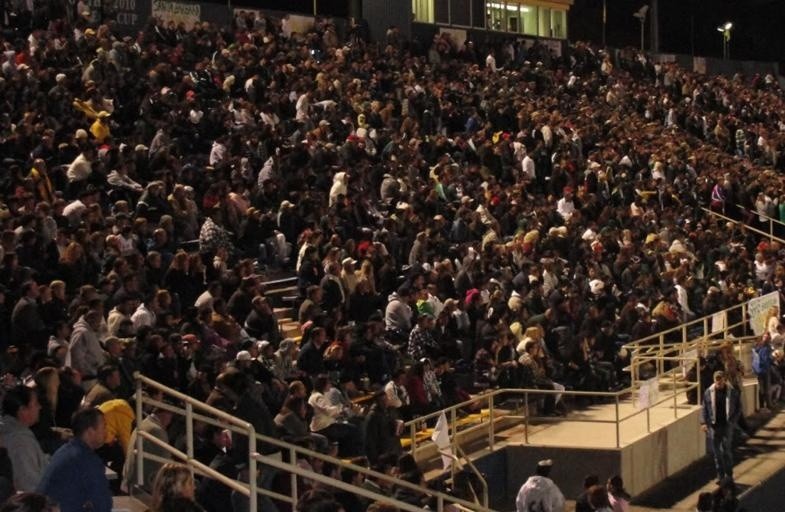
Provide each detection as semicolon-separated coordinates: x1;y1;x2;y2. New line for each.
633;4;649;52
718;22;734;75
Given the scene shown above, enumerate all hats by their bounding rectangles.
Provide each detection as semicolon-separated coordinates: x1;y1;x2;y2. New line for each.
182;335;202;344
281;200;294;209
536;459;552;476
414;232;426;243
151;335;168;348
252;296;266;304
714;370;724;381
585;474;598;485
237;351;256;361
342;257;358;266
257;340;270;352
460;196;475;205
170;333;188;345
443;297;459;308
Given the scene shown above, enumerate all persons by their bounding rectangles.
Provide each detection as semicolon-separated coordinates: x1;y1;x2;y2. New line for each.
0;0;785;512
